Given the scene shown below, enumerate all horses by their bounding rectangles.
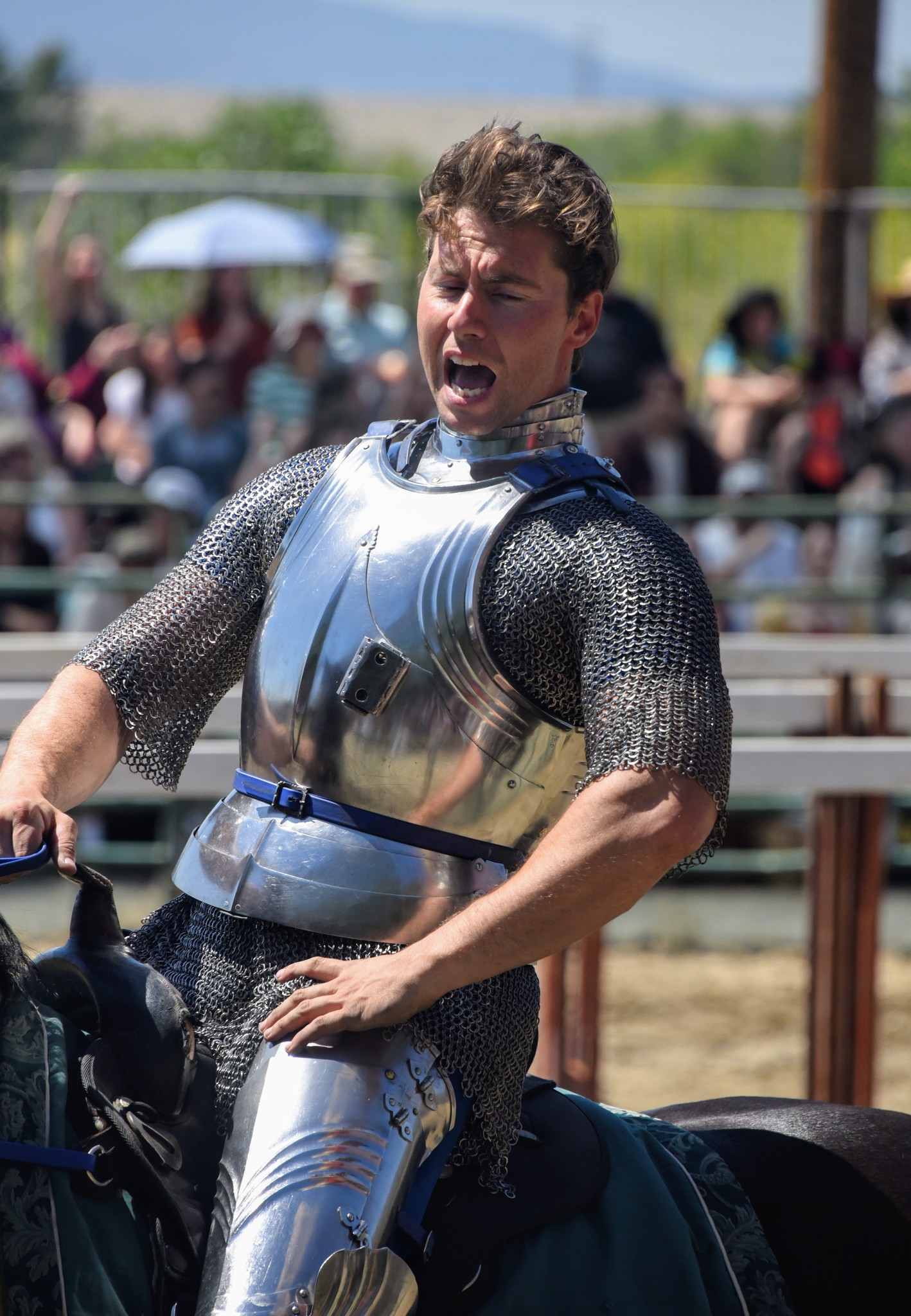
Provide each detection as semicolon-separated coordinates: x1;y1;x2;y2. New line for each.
0;860;911;1316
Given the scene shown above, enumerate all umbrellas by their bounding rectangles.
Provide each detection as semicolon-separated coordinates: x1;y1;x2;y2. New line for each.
112;196;342;273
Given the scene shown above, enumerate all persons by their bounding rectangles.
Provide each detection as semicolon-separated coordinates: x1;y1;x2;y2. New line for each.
0;123;734;1316
4;170;910;664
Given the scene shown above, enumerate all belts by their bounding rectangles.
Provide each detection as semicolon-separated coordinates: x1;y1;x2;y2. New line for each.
233;767;517;870
